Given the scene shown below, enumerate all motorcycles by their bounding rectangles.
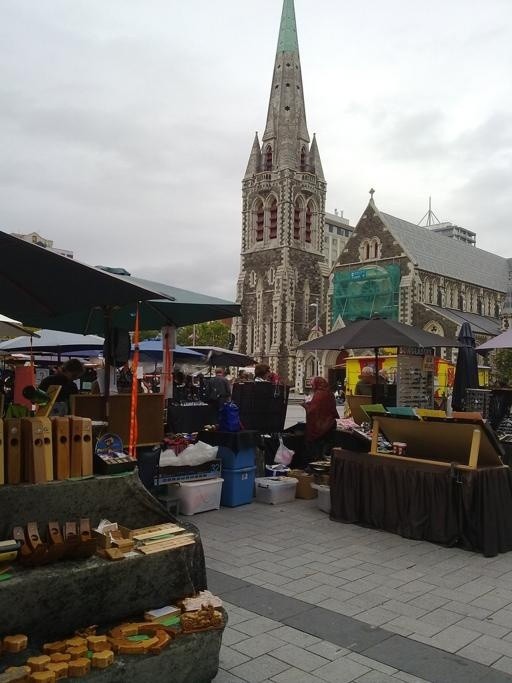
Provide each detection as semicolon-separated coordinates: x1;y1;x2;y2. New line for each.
334;384;346;404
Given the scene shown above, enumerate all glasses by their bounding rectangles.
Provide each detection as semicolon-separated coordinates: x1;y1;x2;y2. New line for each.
463;392;492;418
399;368;432;409
496;412;512;434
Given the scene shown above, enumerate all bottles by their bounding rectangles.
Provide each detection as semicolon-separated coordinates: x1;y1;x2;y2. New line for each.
22;385;49;407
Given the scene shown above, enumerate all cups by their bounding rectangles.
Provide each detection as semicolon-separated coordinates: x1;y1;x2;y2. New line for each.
391;441;407;456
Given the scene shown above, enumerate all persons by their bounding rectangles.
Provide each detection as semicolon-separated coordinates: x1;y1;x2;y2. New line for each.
354;366;374;394
300;374;342;465
1;359;281;412
376;368;389;383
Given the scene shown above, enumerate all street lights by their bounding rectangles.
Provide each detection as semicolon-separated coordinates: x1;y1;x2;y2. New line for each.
309;295;321;378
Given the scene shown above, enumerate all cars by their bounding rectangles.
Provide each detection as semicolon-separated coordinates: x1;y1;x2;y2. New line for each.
0;360;17;396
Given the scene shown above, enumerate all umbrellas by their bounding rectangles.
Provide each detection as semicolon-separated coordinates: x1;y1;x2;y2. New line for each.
290;310;468;376
452;319;480;412
476;324;512;358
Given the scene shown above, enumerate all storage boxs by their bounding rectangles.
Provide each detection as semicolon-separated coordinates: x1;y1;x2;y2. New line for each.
171;430;331;516
